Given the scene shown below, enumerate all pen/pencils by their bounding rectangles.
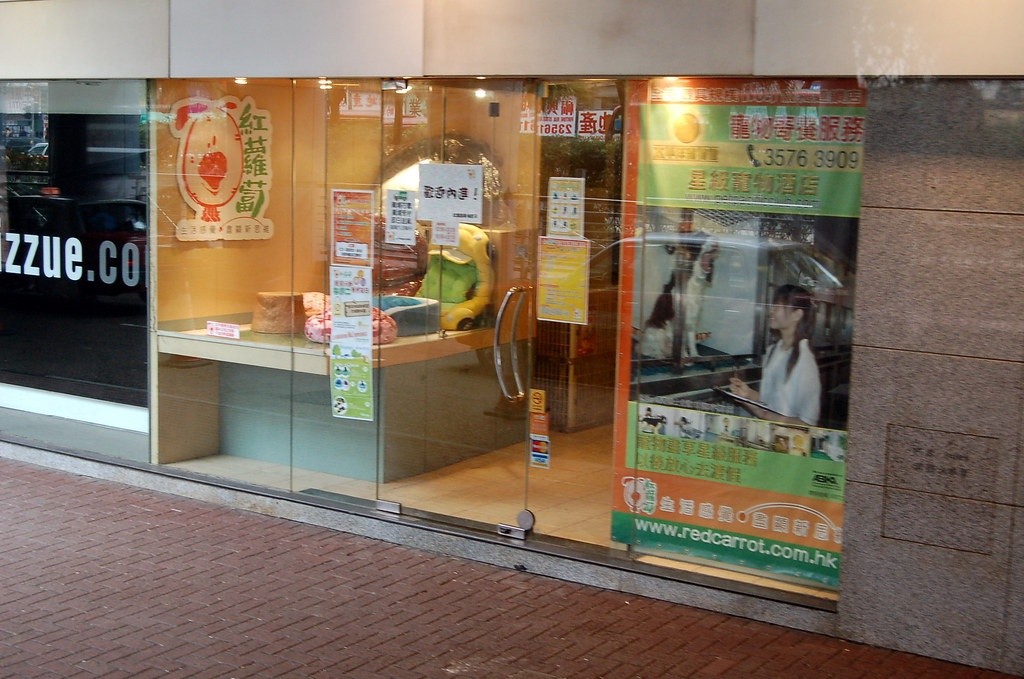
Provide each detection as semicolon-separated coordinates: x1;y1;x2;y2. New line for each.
735;363;740;377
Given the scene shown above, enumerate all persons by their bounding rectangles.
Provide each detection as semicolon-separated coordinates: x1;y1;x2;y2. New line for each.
728;284;822;426
681;416;703;438
645;407;652;417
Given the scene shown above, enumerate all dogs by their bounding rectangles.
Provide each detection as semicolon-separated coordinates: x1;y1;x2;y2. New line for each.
638;415;667;434
634;230;719;368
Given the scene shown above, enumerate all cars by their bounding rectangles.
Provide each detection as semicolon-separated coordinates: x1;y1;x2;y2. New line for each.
60;199;149;306
633;230;845;365
